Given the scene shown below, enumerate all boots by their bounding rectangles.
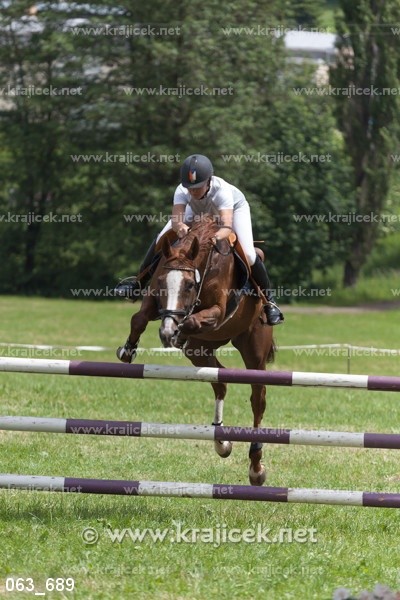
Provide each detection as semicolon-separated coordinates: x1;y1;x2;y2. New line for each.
249;255;282;325
117;238;158;299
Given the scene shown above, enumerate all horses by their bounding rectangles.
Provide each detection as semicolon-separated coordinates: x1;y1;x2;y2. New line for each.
115;211;276;489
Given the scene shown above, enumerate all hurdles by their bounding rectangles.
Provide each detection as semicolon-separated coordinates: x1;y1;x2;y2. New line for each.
0;356;400;510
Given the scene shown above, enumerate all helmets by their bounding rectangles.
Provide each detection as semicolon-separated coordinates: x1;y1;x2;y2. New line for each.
181;154;213;189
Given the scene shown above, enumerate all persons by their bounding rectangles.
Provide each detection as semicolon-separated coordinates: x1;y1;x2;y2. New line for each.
116;154;280;326
